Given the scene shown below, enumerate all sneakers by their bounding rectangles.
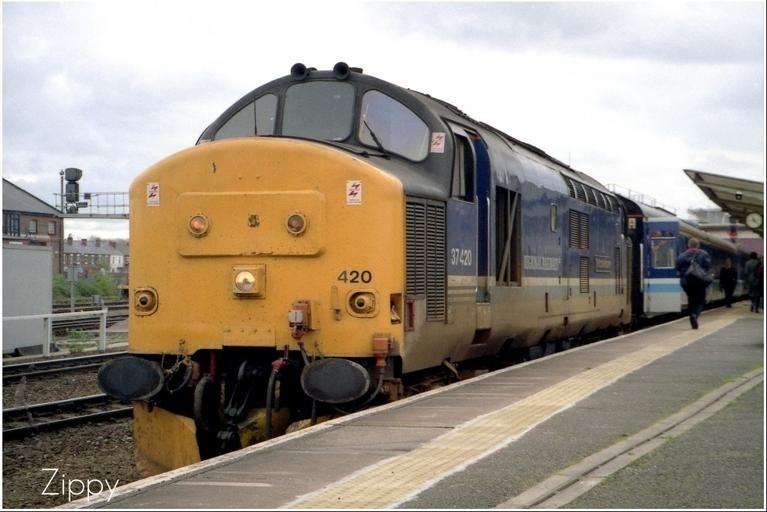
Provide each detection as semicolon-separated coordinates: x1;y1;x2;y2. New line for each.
690;314;698;329
750;304;765;315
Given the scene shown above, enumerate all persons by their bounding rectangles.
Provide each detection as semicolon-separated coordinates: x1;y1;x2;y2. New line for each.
676;237;713;329
743;251;763;312
719;258;738;308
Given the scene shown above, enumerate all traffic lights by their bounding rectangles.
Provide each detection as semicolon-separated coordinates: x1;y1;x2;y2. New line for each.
64;167;82;181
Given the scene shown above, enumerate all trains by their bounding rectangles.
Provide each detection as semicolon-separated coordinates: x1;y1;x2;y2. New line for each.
95;56;763;482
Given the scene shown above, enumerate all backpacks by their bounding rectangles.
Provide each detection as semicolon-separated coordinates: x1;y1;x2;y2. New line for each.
752;261;763;289
684;261;713;289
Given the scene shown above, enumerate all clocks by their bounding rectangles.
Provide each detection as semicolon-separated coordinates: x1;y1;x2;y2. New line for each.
746;213;762;228
